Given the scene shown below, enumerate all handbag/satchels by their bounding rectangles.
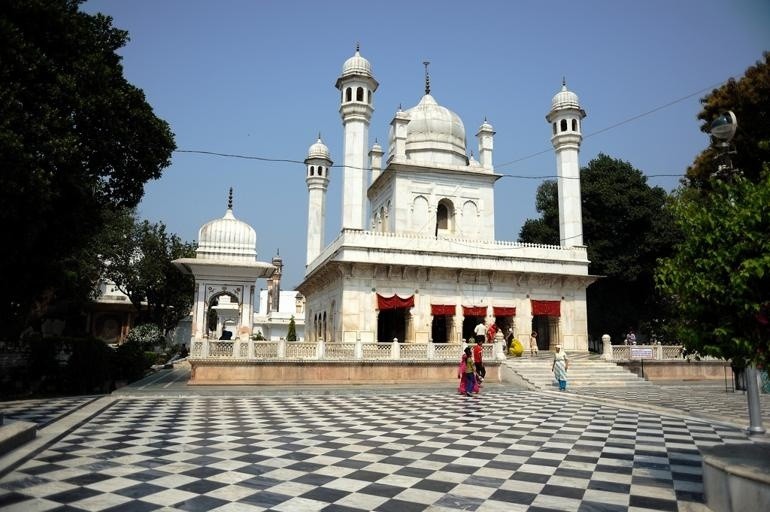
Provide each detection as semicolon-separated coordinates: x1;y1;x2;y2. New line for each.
478;376;484;382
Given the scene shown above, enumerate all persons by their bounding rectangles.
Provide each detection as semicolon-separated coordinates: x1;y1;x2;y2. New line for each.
468;318;514;355
164;343;188;365
465;346;477;396
457;347;481;396
626;327;636;345
473;340;486;388
550;344;569;391
530;330;539;357
509;338;524;357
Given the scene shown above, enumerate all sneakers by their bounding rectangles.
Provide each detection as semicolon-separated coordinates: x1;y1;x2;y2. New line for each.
467;392;472;396
